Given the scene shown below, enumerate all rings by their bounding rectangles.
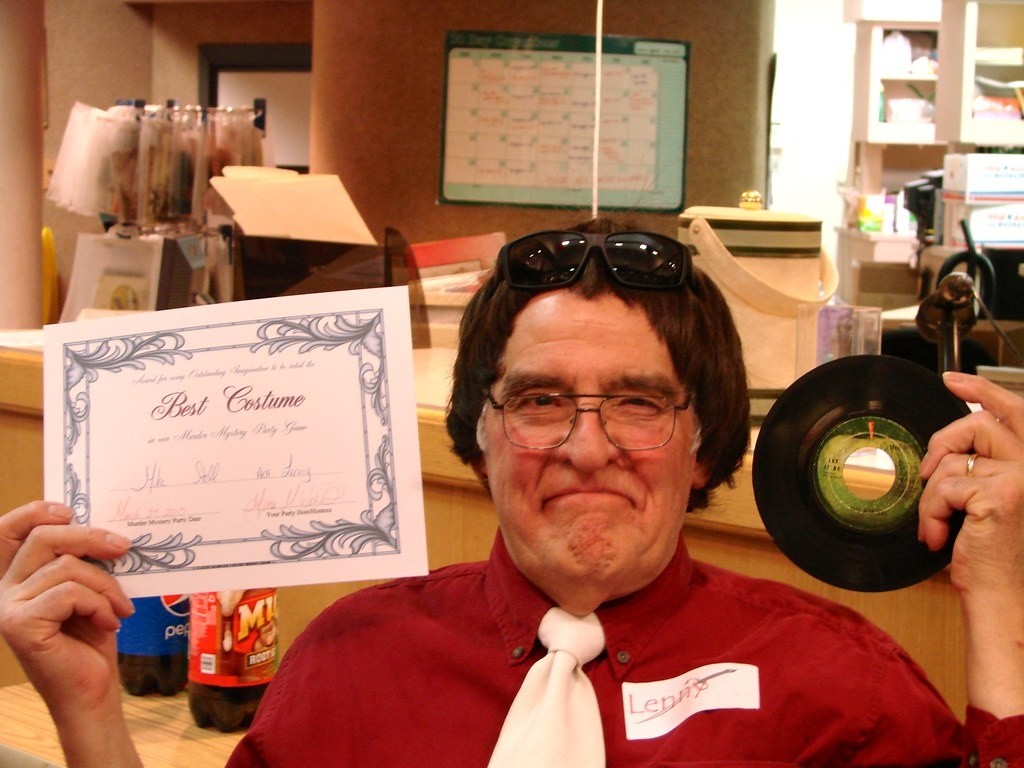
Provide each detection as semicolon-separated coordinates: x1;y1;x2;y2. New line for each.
967;453;980;477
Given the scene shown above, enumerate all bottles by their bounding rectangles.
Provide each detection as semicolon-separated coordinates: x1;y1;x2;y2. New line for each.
187;586;279;734
116;588;189;699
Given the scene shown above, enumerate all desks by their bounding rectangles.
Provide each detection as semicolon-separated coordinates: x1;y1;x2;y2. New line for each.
0;672;251;768
0;322;969;729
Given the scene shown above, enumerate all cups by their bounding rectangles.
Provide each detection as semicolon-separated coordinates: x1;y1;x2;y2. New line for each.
794;298;884;390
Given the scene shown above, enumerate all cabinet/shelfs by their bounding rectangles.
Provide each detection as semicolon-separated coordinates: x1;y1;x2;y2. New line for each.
836;0;1024;314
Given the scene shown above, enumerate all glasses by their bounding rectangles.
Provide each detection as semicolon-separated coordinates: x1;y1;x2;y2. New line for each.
482;229;696;297
482;387;693;450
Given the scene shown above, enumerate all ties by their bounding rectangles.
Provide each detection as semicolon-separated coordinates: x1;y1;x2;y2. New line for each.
486;606;605;768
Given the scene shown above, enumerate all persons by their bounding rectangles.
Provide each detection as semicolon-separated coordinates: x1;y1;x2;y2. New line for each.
0;217;1024;768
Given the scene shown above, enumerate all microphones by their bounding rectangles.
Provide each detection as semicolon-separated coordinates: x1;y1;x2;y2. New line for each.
915;272;977;344
